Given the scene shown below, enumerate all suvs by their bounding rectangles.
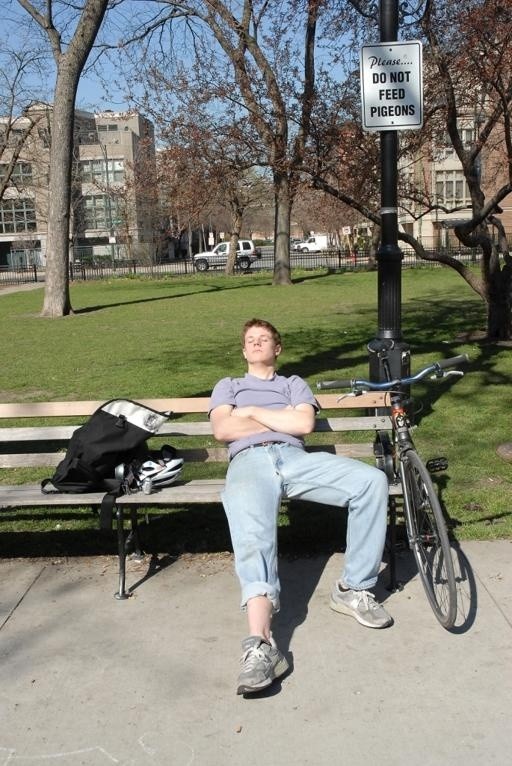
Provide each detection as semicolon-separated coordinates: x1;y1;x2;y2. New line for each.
193;240;261;272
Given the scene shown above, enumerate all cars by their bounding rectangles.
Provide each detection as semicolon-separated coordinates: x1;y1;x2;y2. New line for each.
293;240;301;250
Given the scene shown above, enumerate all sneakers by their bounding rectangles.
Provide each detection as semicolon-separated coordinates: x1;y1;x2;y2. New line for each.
236;635;290;695
330;580;394;629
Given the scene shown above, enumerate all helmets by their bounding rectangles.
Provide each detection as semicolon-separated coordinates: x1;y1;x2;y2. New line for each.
136;457;184;488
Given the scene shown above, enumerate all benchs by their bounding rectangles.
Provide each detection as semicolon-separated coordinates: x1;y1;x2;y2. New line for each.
0;392;405;595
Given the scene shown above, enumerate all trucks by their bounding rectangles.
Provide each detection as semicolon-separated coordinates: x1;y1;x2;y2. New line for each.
297;235;327;253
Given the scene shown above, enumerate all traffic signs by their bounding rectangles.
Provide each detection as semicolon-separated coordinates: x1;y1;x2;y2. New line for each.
360;40;425;132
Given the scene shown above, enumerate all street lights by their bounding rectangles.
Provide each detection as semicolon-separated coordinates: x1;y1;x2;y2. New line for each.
89;132;116;269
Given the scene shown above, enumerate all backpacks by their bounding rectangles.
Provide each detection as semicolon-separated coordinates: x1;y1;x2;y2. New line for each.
41;398;172;495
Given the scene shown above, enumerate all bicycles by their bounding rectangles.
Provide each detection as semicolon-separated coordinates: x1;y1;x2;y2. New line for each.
312;332;470;628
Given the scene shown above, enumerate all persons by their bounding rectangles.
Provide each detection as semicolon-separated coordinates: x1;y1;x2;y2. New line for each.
207;318;394;696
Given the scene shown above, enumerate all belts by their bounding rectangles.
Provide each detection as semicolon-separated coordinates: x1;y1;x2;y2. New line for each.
230;440;285;462
